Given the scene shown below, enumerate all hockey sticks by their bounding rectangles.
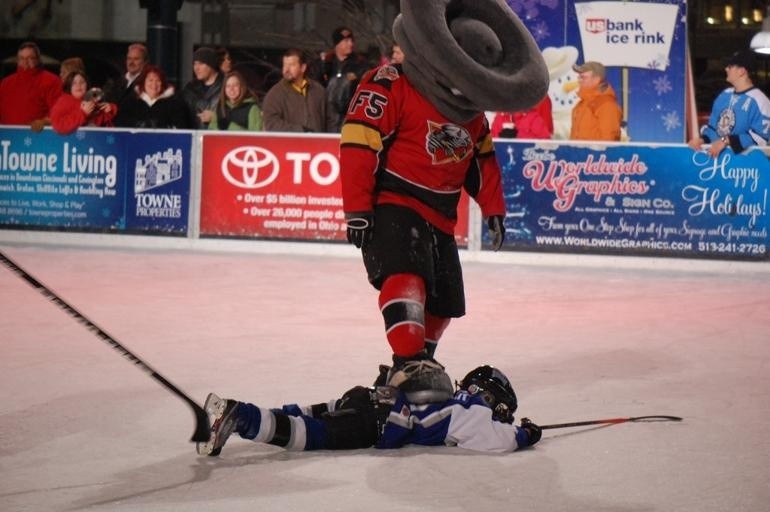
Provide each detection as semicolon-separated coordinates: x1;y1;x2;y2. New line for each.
537;415;683;427
0;251;212;443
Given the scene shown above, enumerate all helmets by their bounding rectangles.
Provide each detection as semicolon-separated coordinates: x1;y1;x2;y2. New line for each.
460;365;519;423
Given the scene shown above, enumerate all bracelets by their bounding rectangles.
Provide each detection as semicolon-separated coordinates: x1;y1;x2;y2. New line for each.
721;136;728;145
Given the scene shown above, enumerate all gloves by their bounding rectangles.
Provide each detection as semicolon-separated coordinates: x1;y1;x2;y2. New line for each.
346;210;374;247
521;417;541;443
488;214;508;251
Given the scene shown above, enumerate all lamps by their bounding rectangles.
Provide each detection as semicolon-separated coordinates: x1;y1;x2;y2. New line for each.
749;12;770;55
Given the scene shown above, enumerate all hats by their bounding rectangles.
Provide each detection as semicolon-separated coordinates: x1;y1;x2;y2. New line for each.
725;51;758;76
193;46;217;68
331;26;354;46
572;62;606;80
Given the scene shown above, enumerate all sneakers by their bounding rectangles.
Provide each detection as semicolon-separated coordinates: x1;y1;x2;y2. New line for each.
209;399;244;457
388;357;454;404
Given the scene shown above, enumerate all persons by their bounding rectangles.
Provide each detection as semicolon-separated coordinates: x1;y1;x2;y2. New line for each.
0;44;336;136
569;62;622;143
337;17;509;402
685;53;769;156
488;93;554;140
193;361;543;457
305;26;404;139
